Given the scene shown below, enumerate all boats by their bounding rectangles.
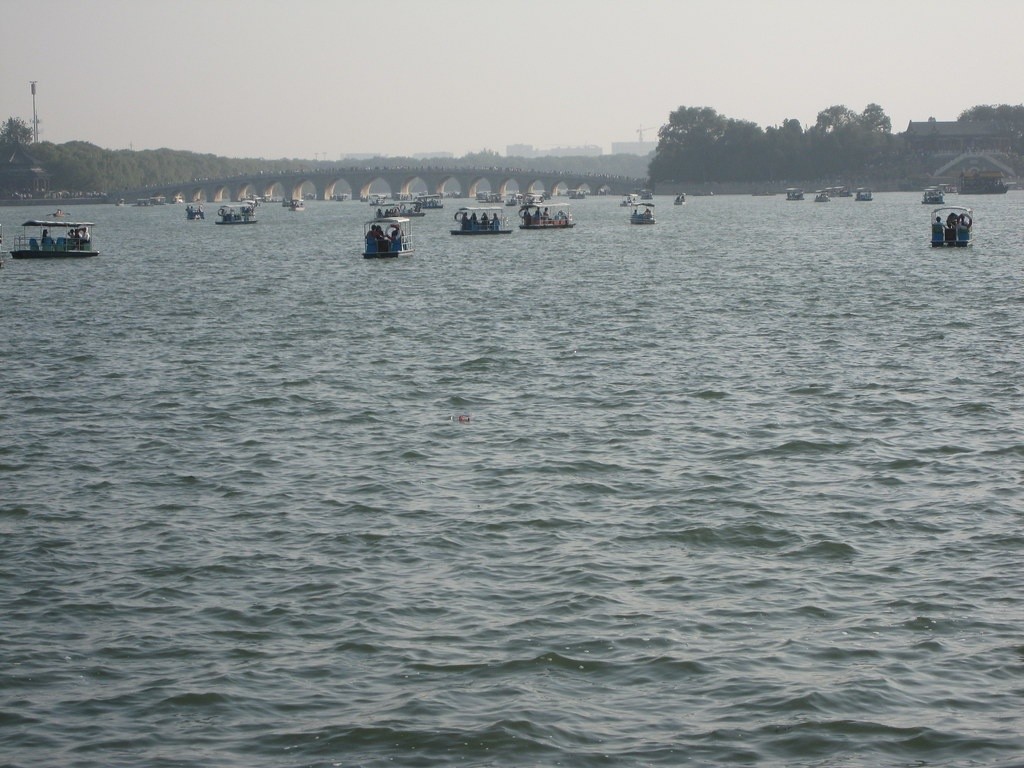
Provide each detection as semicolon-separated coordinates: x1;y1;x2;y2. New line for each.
9;219;100;258
115;200;124;207
930;207;975;246
620;194;640;207
752;190;775;196
449;208;514;234
185;203;207;219
241;194;272;207
921;189;945;205
640;189;653;199
825;187;853;196
362;218;417;258
816;190;830;201
674;197;686;205
938;184;956;192
956;172;1008;194
786;188;805;201
854;188;873;201
629;203;656;224
215;204;258;223
303;193;348;202
475;191;551;206
518;203;576;228
52;213;66;217
133;197;183;207
287;198;305;212
360;193;444;219
565;189;586;199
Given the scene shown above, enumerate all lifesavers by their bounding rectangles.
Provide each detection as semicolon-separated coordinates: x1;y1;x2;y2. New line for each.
518;195;522;199
385;223;402;241
217;209;224;217
75;228;84;238
400;204;406;211
454;211;464;223
518;208;528;218
958;214;972;230
392;206;400;215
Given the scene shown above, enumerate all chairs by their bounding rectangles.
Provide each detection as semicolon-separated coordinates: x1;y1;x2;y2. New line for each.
29;237;65;251
391;237;401;251
470;220;500;230
367;236;378;252
932;224;942;241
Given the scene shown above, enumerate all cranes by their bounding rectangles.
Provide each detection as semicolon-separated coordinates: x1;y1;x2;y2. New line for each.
636;124;658;141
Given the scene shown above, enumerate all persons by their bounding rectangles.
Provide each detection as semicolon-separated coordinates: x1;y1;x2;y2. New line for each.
364;204;421;244
42;227;90;245
933;213;972;231
463;212;499;230
523;207;550;221
633;209;651;217
